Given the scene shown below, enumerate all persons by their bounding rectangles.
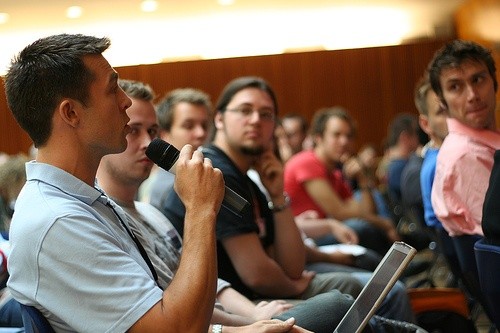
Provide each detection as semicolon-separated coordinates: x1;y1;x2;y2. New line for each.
95;39;500;333
4;33;315;333
0;145;38;332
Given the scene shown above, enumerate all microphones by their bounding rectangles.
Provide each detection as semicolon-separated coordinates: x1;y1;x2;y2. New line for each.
144;138;251;218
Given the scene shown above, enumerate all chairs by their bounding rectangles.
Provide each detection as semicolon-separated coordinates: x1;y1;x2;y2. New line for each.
372;189;500;331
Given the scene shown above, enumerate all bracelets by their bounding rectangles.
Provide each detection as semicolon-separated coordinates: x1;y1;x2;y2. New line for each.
268;193;292;212
212;325;222;332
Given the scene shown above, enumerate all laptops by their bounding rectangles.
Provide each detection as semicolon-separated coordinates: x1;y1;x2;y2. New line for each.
333;241;417;333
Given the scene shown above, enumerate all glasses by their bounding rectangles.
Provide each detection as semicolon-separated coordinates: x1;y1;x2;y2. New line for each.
224;106;277;122
